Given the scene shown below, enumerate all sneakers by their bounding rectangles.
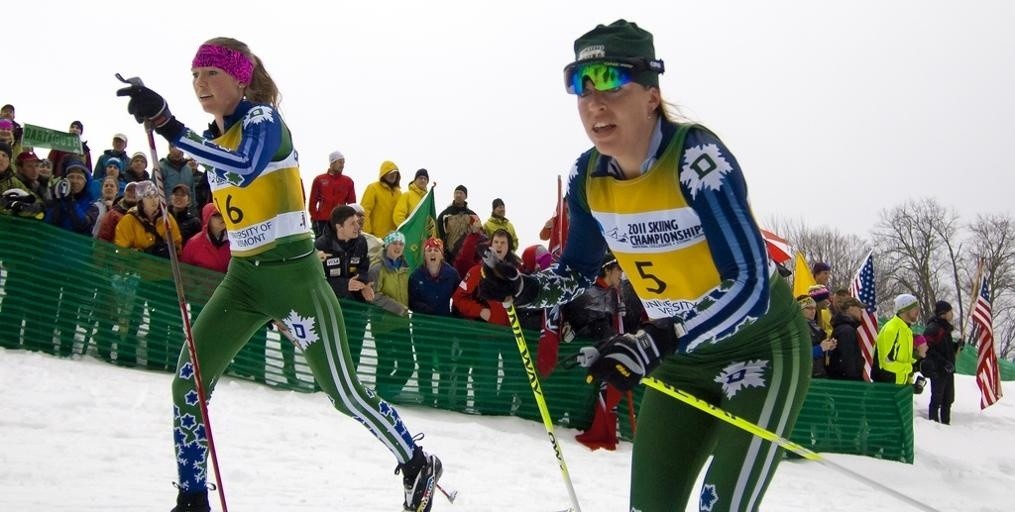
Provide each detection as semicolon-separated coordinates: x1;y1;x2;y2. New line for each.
170;481;216;512
394;433;443;512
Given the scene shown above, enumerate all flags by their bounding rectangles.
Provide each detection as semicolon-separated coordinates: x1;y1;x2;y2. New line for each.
964;271;1003;411
848;255;879;383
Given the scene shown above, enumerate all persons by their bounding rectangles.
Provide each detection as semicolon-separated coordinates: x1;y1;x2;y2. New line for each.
281;151;649;434
922;300;965;425
117;37;444;512
464;20;813;512
796;263;925;450
1;106;232;373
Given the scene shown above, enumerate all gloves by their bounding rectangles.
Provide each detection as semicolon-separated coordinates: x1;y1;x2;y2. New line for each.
479;259;521;302
820;338;834;352
562;323;576;344
587;316;679;392
117;86;184;143
956;339;965;353
944;363;955;373
830;338;837;350
920;358;935;377
616;301;626;316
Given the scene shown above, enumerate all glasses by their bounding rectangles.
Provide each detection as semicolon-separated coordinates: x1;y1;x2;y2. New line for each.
563;55;665;94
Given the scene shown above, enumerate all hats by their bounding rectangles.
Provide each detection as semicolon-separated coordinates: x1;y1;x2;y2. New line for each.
71;121;83;134
66;168;87;182
172;183;191;196
0;143;12;161
808;284;830;302
0;118;13;129
935;301;952;317
415;169;429;183
455;185;467;198
493;198;505;211
536;245;547;256
17;152;43;163
895;293;918;315
840;297;868;309
113;133;127;147
135;180;159;202
812;263;831;278
346;203;365;213
574;19;659;89
424;237;444;256
329;150;345;166
384;231;406;250
104;157;124;172
387;170;399;175
129;152;147;168
124;181;137;192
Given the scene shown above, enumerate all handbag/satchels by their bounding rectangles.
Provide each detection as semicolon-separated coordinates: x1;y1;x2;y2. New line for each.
143;244;169;260
